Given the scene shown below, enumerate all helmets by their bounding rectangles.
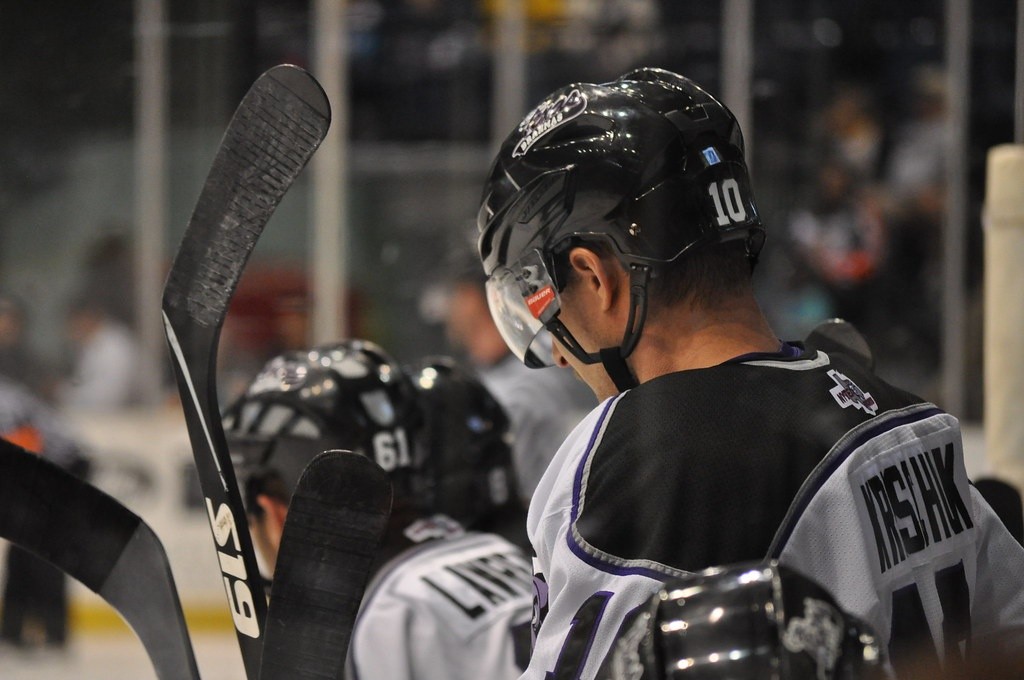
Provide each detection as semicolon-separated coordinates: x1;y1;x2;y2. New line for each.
217;340;434;521
476;69;770;391
400;354;526;519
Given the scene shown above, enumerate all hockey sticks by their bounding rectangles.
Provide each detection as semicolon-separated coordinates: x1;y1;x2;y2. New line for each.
0;440;202;679
160;62;332;679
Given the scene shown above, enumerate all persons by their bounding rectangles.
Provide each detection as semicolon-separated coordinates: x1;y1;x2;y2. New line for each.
479;68;1024;680
221;339;533;679
437;249;599;510
